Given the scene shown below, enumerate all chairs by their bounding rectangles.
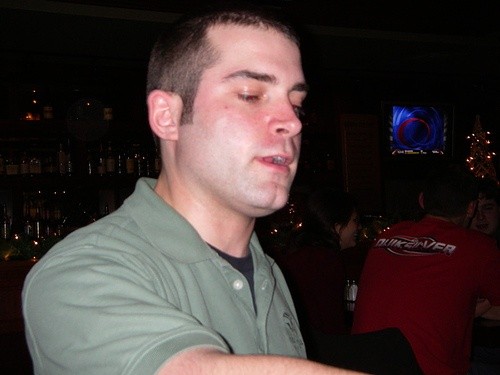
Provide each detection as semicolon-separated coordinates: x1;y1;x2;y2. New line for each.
300;315;423;375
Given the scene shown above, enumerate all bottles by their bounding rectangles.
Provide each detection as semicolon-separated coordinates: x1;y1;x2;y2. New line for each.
0;143;73;176
87;141;149;177
23;207;65;240
0;204;10;239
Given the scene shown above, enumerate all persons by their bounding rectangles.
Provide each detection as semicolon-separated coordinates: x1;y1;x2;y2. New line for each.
352;164;500;375
269;190;363;370
19;4;372;375
466;176;500;367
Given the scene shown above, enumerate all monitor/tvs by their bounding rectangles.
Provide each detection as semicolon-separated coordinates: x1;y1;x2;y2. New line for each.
392;106;444;150
